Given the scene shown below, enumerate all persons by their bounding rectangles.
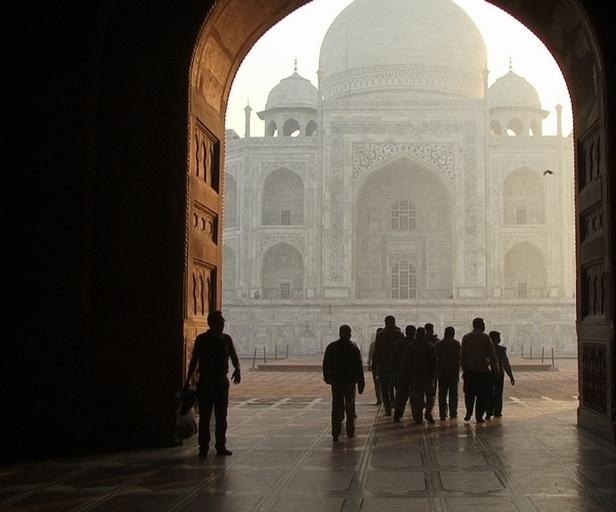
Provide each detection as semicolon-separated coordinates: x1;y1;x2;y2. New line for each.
365;315;514;423
182;310;241;457
321;324;365;441
